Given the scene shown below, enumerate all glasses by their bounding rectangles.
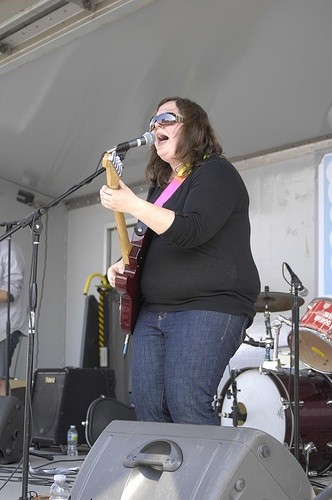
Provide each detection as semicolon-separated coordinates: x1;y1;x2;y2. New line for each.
149;113;186;129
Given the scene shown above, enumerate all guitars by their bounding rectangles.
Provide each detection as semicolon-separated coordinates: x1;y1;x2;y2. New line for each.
98;148;142;336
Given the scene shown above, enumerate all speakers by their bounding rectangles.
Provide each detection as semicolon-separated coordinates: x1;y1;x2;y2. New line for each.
68;419;317;500
29;367;115;446
0;395;25;464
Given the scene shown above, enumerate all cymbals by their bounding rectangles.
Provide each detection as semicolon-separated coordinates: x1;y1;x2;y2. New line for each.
257;289;306;314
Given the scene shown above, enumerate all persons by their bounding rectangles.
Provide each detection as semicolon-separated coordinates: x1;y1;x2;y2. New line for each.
98;96;262;426
0;234;31;384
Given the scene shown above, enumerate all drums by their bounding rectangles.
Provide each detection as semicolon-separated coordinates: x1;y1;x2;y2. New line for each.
285;295;332;374
218;362;331;482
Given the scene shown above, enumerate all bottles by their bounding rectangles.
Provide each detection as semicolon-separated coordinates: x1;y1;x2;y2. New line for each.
68;425;78;456
49;475;70;500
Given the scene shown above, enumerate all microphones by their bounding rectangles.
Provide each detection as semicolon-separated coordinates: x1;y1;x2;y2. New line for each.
244;341;274;349
284;262;308;297
106;132;156;154
